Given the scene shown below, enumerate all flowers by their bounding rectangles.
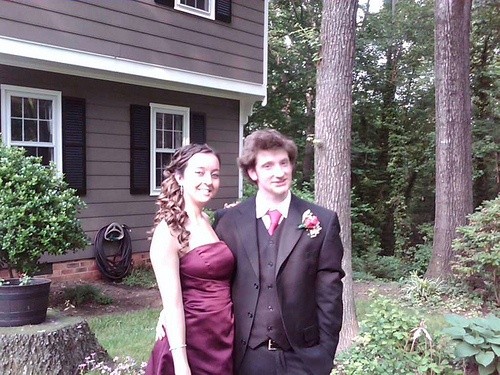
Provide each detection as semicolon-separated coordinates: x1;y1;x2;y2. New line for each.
299;209;322;238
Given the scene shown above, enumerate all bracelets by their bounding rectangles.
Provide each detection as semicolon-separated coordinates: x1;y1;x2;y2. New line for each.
169;344;187;352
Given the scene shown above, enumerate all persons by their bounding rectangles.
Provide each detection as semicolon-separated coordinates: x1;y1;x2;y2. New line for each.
155;128;346;375
145;144;237;375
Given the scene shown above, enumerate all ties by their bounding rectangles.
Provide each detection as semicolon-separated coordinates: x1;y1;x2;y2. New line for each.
266;209;282;236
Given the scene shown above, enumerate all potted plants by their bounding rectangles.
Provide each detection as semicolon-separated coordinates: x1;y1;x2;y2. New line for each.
0;136;90;327
